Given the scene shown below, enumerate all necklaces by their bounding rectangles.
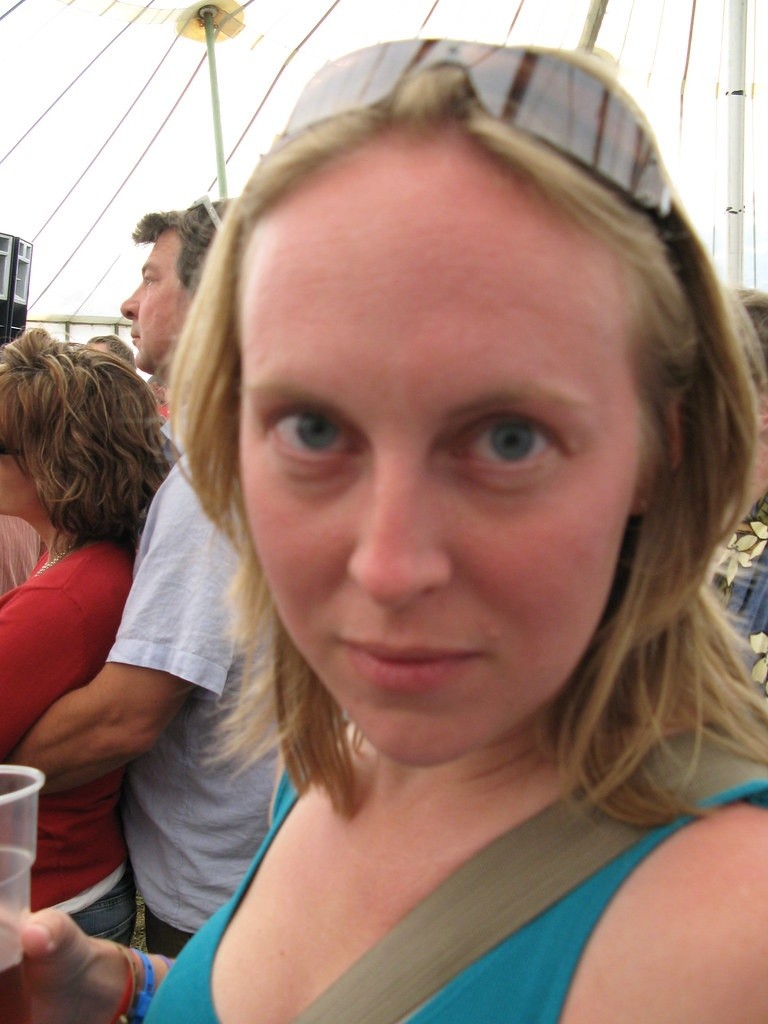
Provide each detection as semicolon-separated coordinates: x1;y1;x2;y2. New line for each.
32;545;72;577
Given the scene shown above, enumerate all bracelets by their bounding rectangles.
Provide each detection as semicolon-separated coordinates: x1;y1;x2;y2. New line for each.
111;943;154;1024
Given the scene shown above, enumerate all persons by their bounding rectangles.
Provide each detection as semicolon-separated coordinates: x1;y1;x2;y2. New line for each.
0;201;768;950
0;38;768;1024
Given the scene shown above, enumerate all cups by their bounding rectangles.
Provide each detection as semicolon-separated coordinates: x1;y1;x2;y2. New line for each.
0;764;45;972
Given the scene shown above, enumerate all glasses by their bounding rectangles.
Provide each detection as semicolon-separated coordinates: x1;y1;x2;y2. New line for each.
285;39;696;302
189;194;222;235
